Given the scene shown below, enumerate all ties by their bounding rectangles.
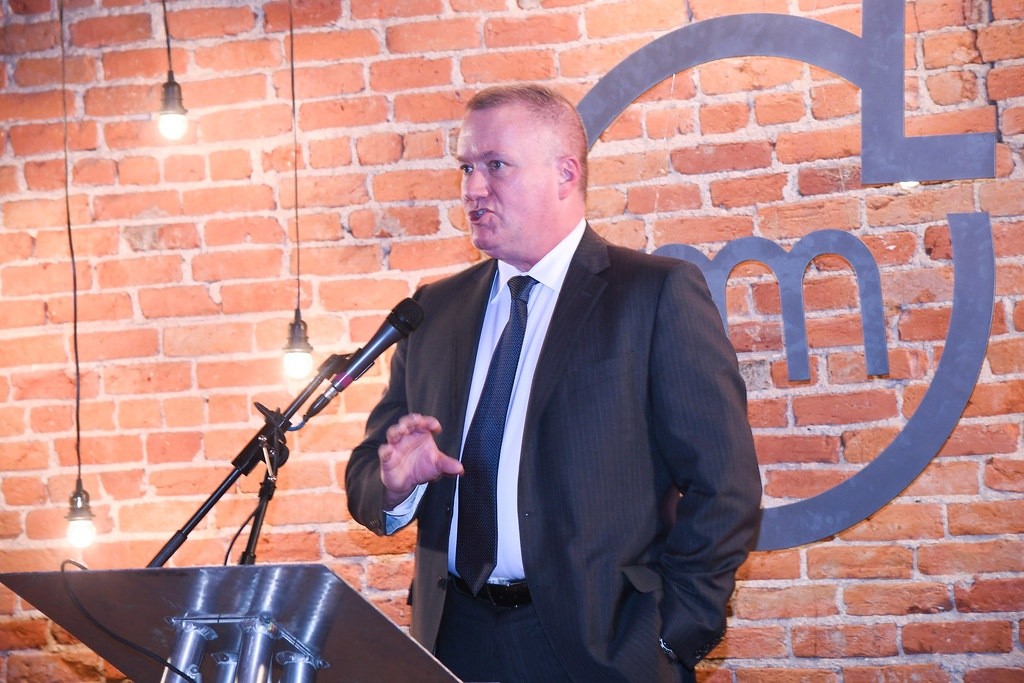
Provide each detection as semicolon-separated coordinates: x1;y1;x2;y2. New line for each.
454;276;543;595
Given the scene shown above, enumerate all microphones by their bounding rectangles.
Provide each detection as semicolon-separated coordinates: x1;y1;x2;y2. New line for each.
302;297;425;423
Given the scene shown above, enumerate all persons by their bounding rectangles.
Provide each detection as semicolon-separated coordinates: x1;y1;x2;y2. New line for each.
341;81;765;680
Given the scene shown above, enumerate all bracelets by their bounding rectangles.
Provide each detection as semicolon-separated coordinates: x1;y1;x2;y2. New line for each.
659;636;675;658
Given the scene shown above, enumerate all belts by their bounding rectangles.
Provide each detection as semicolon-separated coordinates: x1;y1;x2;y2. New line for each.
445;571;535;611
